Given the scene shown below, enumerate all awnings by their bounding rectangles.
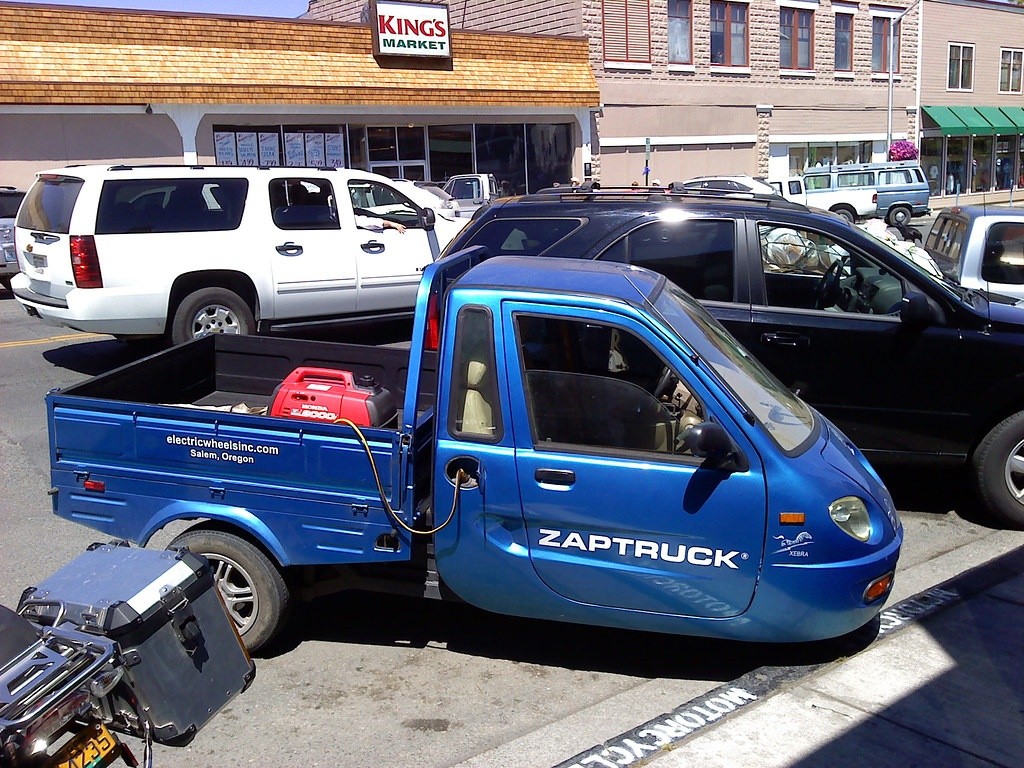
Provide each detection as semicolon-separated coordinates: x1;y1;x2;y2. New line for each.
921;105;1024;137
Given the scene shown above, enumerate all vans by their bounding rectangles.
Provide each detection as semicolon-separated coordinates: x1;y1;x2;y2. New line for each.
800;160;932;226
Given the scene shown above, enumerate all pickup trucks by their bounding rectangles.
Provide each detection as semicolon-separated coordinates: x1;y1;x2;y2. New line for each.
762;205;1024;325
762;177;878;225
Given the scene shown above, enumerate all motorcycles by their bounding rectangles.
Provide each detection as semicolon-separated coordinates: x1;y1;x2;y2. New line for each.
1;537;256;768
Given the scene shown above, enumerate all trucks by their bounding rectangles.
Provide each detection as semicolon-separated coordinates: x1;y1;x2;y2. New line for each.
440;174;508;209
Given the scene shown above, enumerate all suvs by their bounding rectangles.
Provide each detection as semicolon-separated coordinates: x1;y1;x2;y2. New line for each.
347;180;461;218
9;156;473;348
0;186;28;292
426;191;1024;526
663;175;776;200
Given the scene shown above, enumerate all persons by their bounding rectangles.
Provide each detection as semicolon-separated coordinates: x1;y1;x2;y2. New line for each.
652;179;661;186
631;182;641;192
569;177;580;187
354;214;407;235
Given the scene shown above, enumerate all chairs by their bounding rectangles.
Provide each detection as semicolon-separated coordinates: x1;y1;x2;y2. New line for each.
704;270;732;302
982;270;1002;283
108;185;241;233
456;341;496;436
